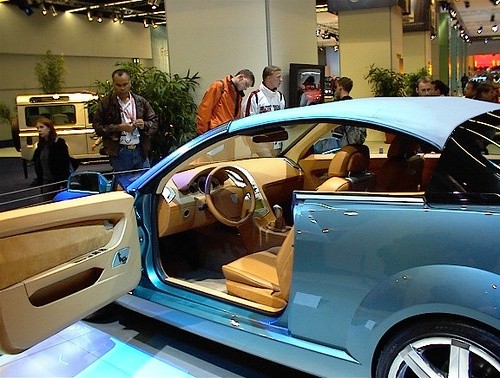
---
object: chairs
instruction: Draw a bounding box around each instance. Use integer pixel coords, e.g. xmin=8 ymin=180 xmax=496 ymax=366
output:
xmin=365 ymin=134 xmax=425 ymax=192
xmin=222 ymin=143 xmax=375 ymax=310
xmin=28 ymin=115 xmax=45 ymax=127
xmin=51 ymin=113 xmax=69 ymax=125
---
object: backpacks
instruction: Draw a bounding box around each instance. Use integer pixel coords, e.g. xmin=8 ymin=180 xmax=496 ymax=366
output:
xmin=339 ymin=125 xmax=367 ymax=147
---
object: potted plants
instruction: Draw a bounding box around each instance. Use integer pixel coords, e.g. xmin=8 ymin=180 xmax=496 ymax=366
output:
xmin=0 ymin=104 xmax=32 ymax=152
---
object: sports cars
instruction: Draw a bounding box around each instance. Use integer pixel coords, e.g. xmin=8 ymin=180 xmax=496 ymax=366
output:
xmin=0 ymin=98 xmax=500 ymax=378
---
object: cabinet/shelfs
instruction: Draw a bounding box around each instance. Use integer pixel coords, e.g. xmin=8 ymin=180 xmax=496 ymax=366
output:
xmin=289 ymin=63 xmax=325 ymax=108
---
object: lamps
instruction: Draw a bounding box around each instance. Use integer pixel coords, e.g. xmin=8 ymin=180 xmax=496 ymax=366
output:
xmin=40 ymin=3 xmax=48 ymax=16
xmin=49 ymin=5 xmax=58 ymax=18
xmin=332 ymin=43 xmax=339 ymax=52
xmin=316 ymin=23 xmax=339 ymax=42
xmin=113 ymin=13 xmax=119 ymax=22
xmin=319 ymin=46 xmax=326 ymax=51
xmin=24 ymin=5 xmax=34 ymax=16
xmin=120 ymin=14 xmax=125 ymax=24
xmin=151 ymin=19 xmax=158 ymax=29
xmin=143 ymin=18 xmax=150 ymax=28
xmin=87 ymin=10 xmax=94 ymax=22
xmin=96 ymin=16 xmax=104 ymax=24
xmin=147 ymin=0 xmax=162 ymax=10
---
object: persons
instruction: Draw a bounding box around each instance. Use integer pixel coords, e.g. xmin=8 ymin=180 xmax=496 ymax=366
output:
xmin=197 ymin=69 xmax=255 ymax=136
xmin=336 ymin=76 xmax=354 ymax=101
xmin=240 ymin=66 xmax=286 ymax=119
xmin=32 ymin=117 xmax=75 ymax=202
xmin=300 ymin=75 xmax=323 ymax=107
xmin=415 ymin=65 xmax=500 ymax=104
xmin=91 ymin=68 xmax=159 ymax=173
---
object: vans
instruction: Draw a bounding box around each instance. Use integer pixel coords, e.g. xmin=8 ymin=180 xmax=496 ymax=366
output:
xmin=17 ymin=90 xmax=174 ymax=160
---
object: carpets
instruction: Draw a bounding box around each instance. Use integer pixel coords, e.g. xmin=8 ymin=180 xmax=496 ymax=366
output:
xmin=0 ymin=157 xmax=65 ymax=213
